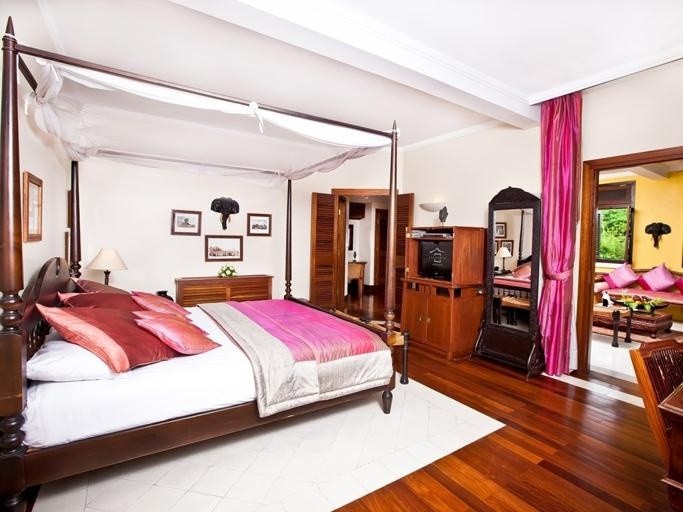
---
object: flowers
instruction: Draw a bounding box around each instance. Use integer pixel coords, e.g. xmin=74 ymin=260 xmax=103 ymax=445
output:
xmin=218 ymin=264 xmax=237 ymax=277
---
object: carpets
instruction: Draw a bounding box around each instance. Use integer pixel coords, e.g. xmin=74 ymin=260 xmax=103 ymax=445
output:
xmin=593 ymin=325 xmax=683 ymax=343
xmin=31 ymin=371 xmax=507 ymax=512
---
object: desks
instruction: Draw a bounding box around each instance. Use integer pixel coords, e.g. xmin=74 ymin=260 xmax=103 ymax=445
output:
xmin=657 ymin=381 xmax=683 ymax=512
xmin=348 ymin=262 xmax=367 ymax=305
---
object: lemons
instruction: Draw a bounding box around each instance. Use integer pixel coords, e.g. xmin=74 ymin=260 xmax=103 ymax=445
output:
xmin=628 ymin=298 xmax=634 ymax=303
xmin=635 ymin=301 xmax=642 ymax=305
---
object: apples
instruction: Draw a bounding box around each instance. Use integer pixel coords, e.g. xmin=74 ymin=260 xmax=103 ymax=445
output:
xmin=633 ymin=294 xmax=641 ymax=301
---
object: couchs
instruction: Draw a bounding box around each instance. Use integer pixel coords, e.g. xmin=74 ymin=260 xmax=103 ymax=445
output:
xmin=594 ymin=267 xmax=683 ymax=322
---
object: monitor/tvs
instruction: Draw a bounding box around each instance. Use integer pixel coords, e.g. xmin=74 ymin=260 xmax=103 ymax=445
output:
xmin=418 ymin=240 xmax=452 ymax=281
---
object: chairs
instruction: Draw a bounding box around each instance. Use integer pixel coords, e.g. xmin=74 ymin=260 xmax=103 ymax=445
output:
xmin=629 ymin=339 xmax=683 ymax=477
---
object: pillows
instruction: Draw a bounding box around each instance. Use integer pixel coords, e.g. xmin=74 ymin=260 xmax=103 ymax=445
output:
xmin=675 ymin=276 xmax=683 ymax=296
xmin=27 ymin=275 xmax=222 ymax=382
xmin=636 ymin=263 xmax=680 ymax=292
xmin=602 ymin=262 xmax=639 ymax=289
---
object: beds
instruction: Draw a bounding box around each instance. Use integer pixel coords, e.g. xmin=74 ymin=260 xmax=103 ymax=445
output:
xmin=0 ymin=17 xmax=398 ymax=512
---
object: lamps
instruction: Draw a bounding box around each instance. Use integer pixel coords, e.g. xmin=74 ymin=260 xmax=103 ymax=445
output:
xmin=495 ymin=247 xmax=512 ymax=272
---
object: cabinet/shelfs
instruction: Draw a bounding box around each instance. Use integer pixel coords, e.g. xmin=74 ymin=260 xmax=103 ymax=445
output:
xmin=400 ymin=226 xmax=487 ymax=361
xmin=175 ymin=275 xmax=273 ymax=307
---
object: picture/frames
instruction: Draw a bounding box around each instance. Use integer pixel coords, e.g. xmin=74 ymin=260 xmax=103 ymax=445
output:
xmin=23 ymin=171 xmax=43 ymax=243
xmin=495 ymin=223 xmax=513 ymax=256
xmin=349 ymin=225 xmax=353 ymax=250
xmin=171 ymin=209 xmax=202 ymax=236
xmin=205 ymin=235 xmax=243 ymax=262
xmin=248 ymin=212 xmax=271 ymax=236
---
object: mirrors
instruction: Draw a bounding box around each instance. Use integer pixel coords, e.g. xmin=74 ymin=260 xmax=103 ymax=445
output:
xmin=468 ymin=185 xmax=545 ymax=383
